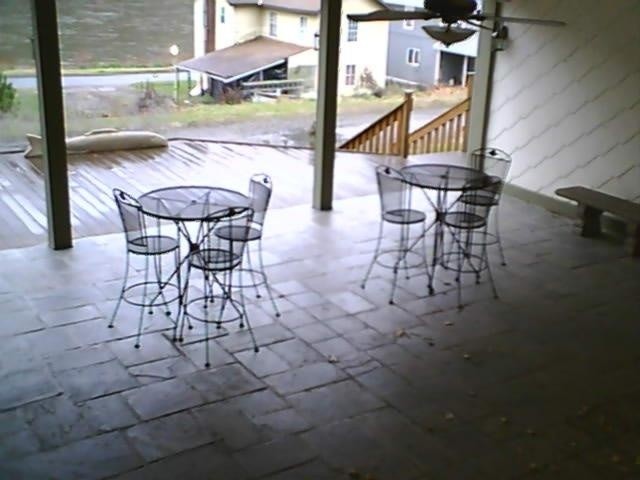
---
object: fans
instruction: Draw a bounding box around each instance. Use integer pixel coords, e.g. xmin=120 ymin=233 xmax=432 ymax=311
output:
xmin=346 ymin=0 xmax=567 ymax=34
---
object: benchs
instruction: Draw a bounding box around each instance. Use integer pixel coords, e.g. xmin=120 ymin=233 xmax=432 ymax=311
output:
xmin=554 ymin=186 xmax=640 ymax=257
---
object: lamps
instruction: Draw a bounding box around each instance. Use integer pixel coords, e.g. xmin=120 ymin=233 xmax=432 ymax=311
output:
xmin=491 ymin=26 xmax=508 ymax=52
xmin=421 ymin=16 xmax=476 ymax=48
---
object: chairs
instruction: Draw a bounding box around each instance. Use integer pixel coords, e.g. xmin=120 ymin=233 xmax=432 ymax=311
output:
xmin=360 ymin=147 xmax=512 ymax=309
xmin=108 ymin=172 xmax=281 ymax=368
